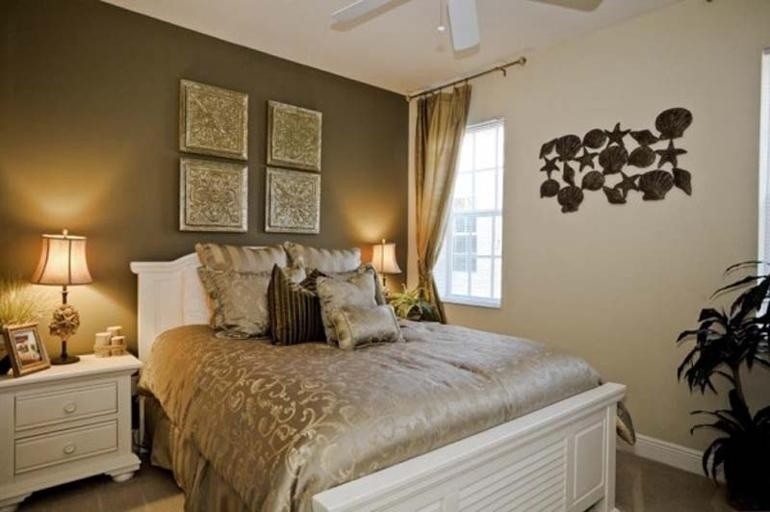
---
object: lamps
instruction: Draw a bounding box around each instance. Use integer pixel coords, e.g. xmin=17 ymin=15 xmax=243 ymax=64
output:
xmin=28 ymin=228 xmax=96 ymax=367
xmin=367 ymin=238 xmax=402 ymax=296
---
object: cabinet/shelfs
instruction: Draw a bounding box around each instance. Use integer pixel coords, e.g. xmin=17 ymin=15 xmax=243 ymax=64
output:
xmin=0 ymin=348 xmax=142 ymax=512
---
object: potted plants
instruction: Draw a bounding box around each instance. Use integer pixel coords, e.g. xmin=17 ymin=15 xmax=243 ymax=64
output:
xmin=669 ymin=259 xmax=770 ymax=507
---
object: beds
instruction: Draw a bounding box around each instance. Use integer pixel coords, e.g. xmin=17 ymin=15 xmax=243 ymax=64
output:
xmin=126 ymin=247 xmax=628 ymax=512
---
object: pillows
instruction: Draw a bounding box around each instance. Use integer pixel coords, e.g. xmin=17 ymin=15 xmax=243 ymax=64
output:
xmin=192 ymin=243 xmax=404 ymax=351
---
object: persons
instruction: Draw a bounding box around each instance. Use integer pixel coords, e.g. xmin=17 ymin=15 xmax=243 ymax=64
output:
xmin=22 ymin=344 xmax=32 ymax=360
xmin=15 ymin=343 xmax=23 ymax=360
xmin=27 ymin=344 xmax=40 ymax=360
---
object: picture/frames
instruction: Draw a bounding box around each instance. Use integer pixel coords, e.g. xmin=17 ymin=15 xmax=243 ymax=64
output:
xmin=4 ymin=323 xmax=51 ymax=377
